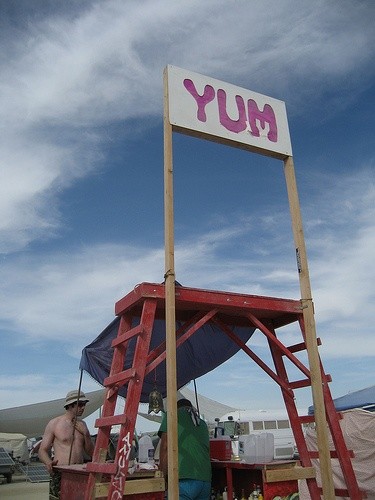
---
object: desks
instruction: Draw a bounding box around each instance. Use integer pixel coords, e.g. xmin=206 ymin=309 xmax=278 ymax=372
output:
xmin=53 ymin=464 xmax=165 ymax=500
xmin=213 ymin=460 xmax=299 ymax=500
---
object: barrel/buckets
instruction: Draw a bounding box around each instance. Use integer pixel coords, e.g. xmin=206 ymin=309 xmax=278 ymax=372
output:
xmin=238 ymin=433 xmax=274 ymax=463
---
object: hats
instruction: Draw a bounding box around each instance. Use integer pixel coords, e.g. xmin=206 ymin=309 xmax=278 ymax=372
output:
xmin=64 ymin=390 xmax=90 ymax=408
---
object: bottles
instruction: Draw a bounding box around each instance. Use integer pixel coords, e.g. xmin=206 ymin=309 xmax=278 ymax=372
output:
xmin=138 ymin=434 xmax=154 ymax=463
xmin=211 ymin=485 xmax=263 ymax=500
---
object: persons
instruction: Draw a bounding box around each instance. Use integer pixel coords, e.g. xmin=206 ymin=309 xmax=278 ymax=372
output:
xmin=128 ymin=428 xmax=139 ymax=469
xmin=157 ymin=398 xmax=213 ymax=500
xmin=37 ymin=389 xmax=93 ymax=500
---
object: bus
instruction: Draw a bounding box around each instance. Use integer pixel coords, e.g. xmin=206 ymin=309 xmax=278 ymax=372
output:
xmin=215 ymin=409 xmax=305 ymax=459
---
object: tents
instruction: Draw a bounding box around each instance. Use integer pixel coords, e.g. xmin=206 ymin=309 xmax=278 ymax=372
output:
xmin=0 ymin=281 xmax=246 ymax=440
xmin=307 ymin=385 xmax=375 ymax=416
